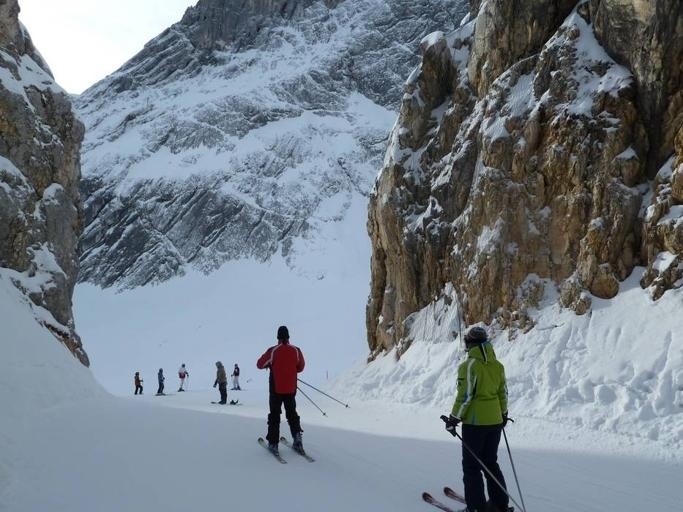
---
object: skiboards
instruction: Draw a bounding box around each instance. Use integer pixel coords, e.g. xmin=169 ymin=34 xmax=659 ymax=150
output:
xmin=151 ymin=393 xmax=176 ymax=396
xmin=227 ymin=388 xmax=246 ymax=391
xmin=258 ymin=437 xmax=315 ymax=464
xmin=211 ymin=399 xmax=238 ymax=405
xmin=422 ymin=487 xmax=514 ymax=512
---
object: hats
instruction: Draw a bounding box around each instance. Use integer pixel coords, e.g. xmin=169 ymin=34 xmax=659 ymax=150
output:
xmin=464 ymin=327 xmax=487 ymax=347
xmin=277 ymin=326 xmax=290 ymax=338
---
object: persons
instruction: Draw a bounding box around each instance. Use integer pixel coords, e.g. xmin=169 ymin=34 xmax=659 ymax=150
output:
xmin=135 ymin=372 xmax=143 ymax=395
xmin=178 ymin=363 xmax=189 ymax=391
xmin=213 ymin=362 xmax=227 ymax=404
xmin=157 ymin=368 xmax=165 ymax=394
xmin=231 ymin=364 xmax=240 ymax=390
xmin=447 ymin=328 xmax=508 ymax=511
xmin=257 ymin=326 xmax=305 ymax=452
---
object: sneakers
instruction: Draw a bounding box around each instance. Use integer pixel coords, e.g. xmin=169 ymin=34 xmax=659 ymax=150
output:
xmin=266 ymin=442 xmax=278 ymax=451
xmin=486 ymin=500 xmax=509 ymax=512
xmin=293 ymin=436 xmax=302 ymax=447
xmin=458 ymin=507 xmax=488 ymax=512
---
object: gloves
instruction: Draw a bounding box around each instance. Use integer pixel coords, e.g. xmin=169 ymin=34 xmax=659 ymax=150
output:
xmin=446 ymin=413 xmax=461 ymax=432
xmin=501 ymin=411 xmax=509 ymax=428
